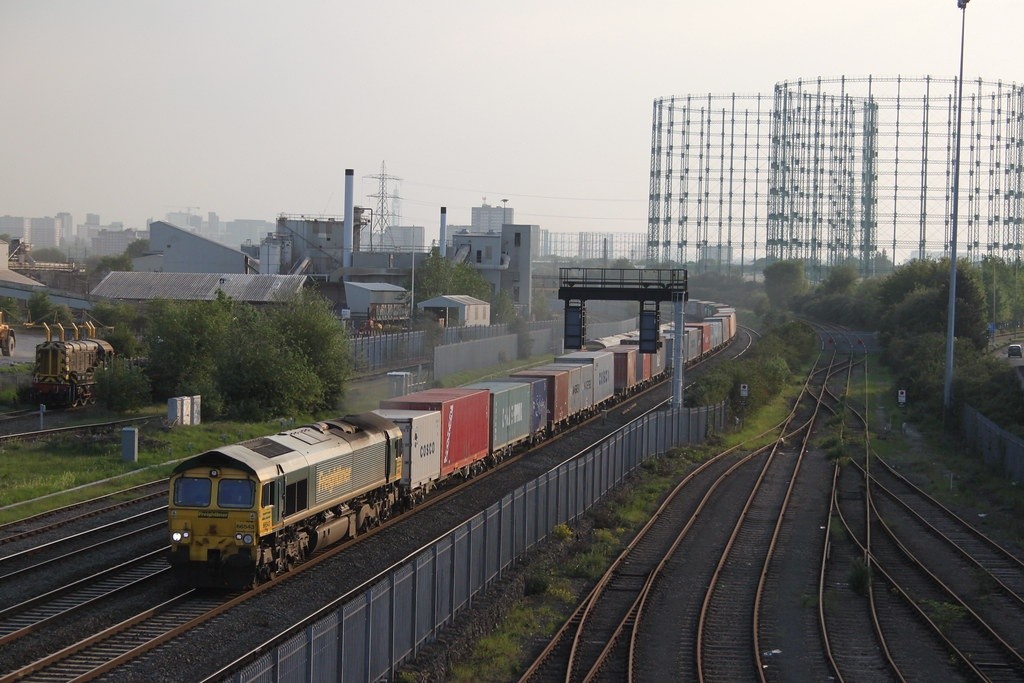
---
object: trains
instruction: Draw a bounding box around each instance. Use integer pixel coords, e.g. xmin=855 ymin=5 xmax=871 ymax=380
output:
xmin=166 ymin=297 xmax=738 ymax=595
xmin=35 ymin=337 xmax=149 ymax=408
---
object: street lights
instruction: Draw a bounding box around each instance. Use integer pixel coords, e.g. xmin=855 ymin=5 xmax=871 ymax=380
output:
xmin=500 ymin=199 xmax=508 ymax=224
xmin=945 ymin=0 xmax=968 ymax=403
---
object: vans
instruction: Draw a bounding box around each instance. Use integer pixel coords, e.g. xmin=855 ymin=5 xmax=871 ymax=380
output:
xmin=1008 ymin=344 xmax=1022 ymax=358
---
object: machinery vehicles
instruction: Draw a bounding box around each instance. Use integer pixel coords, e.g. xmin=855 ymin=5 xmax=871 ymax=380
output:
xmin=0 ymin=312 xmax=17 ymax=356
